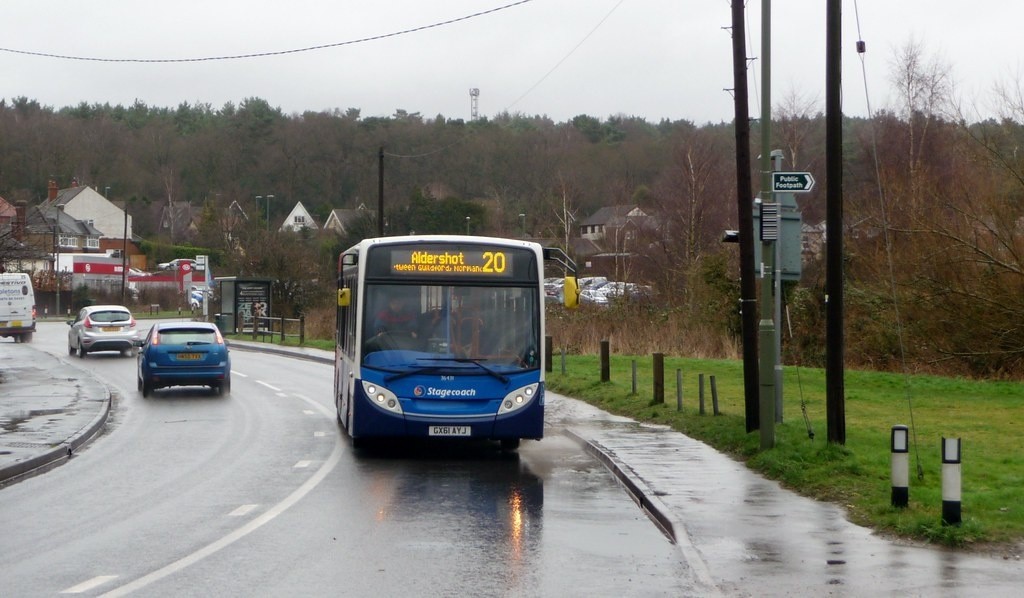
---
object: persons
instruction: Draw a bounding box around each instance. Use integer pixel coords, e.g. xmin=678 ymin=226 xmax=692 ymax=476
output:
xmin=375 ymin=294 xmax=418 ymax=339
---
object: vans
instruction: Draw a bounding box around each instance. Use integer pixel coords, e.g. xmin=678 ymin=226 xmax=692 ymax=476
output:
xmin=0 ymin=273 xmax=36 ymax=343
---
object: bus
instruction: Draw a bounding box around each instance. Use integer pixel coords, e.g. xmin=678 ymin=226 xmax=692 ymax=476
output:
xmin=334 ymin=234 xmax=580 ymax=458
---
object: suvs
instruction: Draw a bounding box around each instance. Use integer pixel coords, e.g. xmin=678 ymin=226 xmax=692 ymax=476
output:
xmin=158 ymin=258 xmax=196 ymax=272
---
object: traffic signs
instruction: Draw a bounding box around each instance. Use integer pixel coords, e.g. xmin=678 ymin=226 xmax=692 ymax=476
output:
xmin=771 ymin=170 xmax=815 ymax=193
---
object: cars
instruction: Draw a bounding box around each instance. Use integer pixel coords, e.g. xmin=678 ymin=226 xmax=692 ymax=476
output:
xmin=133 ymin=320 xmax=232 ymax=400
xmin=544 ymin=276 xmax=652 ymax=307
xmin=129 ymin=266 xmax=154 ymax=277
xmin=66 ymin=306 xmax=137 ymax=358
xmin=191 ymin=286 xmax=213 ymax=310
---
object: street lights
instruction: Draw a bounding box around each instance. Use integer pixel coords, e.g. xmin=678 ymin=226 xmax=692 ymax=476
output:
xmin=104 ymin=187 xmax=111 ymax=199
xmin=518 ymin=213 xmax=525 ymax=239
xmin=466 ymin=216 xmax=470 ymax=235
xmin=254 ymin=195 xmax=263 ymax=231
xmin=757 ymin=150 xmax=784 ymax=424
xmin=53 ymin=203 xmax=66 ymax=316
xmin=266 ymin=194 xmax=275 ymax=233
